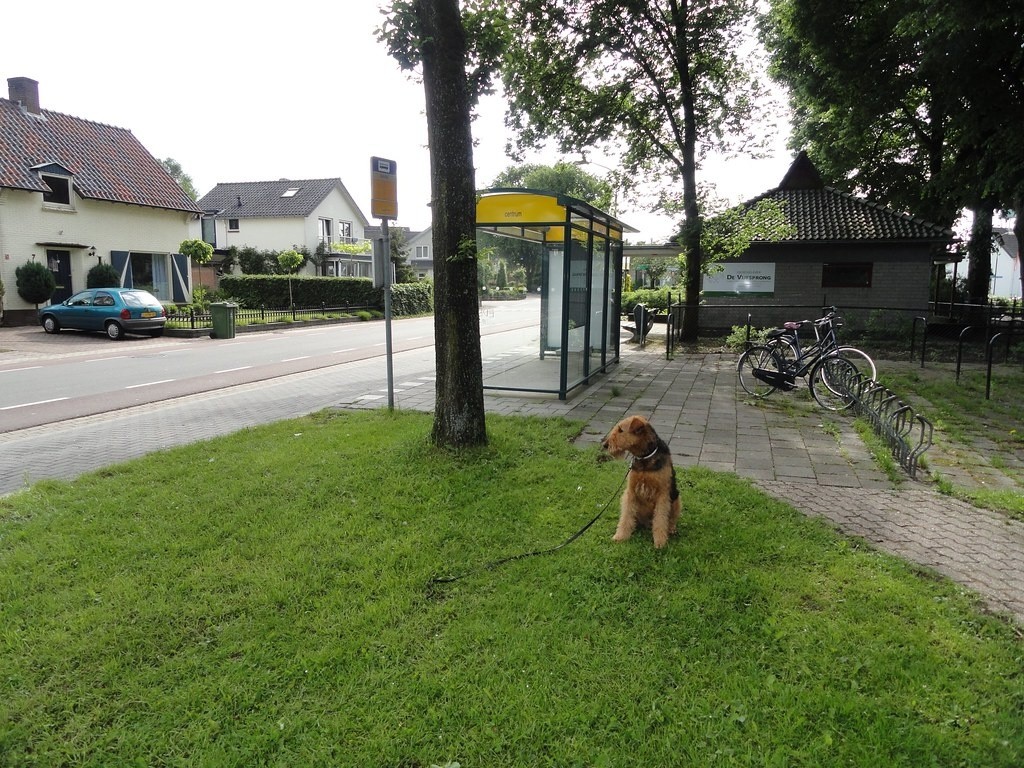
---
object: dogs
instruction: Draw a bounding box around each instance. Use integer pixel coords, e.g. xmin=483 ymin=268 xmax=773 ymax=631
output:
xmin=603 ymin=415 xmax=680 ymax=549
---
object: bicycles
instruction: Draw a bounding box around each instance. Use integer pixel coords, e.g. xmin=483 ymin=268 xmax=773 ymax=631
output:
xmin=736 ymin=306 xmax=861 ymax=411
xmin=759 ymin=317 xmax=878 ymax=398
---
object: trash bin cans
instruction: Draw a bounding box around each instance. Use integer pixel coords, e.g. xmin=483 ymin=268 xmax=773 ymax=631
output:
xmin=209 ymin=301 xmax=237 ymax=338
xmin=672 ymin=302 xmax=687 ymax=330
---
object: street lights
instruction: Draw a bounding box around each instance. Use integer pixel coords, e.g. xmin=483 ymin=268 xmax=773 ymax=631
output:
xmin=573 ymin=160 xmax=618 ymax=218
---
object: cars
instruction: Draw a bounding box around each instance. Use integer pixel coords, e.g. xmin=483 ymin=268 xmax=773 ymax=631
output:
xmin=37 ymin=287 xmax=166 ymax=340
